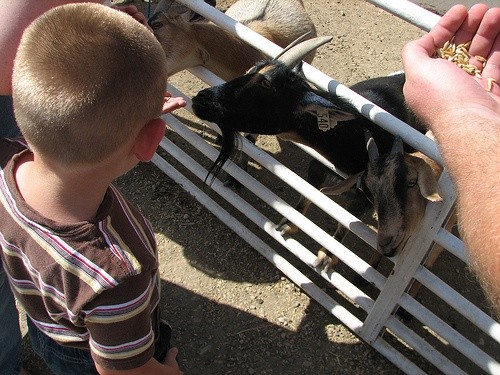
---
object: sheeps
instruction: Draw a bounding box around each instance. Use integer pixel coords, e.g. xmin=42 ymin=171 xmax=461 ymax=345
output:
xmin=190 ymin=31 xmax=432 ymax=274
xmin=318 ymin=128 xmax=458 ymax=318
xmin=146 ymin=0 xmax=317 ymax=190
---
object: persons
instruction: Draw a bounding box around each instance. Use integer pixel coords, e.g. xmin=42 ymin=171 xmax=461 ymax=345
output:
xmin=0 ymin=0 xmax=186 ymax=375
xmin=402 ymin=2 xmax=499 ymax=323
